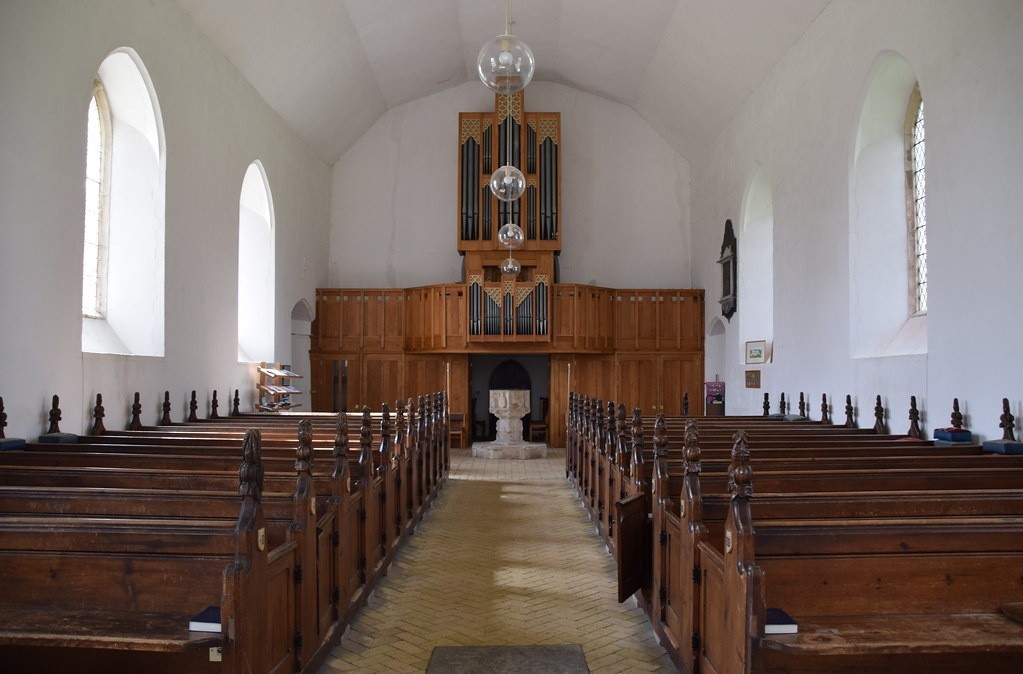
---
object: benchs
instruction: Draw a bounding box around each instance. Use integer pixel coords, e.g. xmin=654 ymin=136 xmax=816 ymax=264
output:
xmin=563 ymin=393 xmax=1023 ymax=674
xmin=0 ymin=389 xmax=450 ymax=674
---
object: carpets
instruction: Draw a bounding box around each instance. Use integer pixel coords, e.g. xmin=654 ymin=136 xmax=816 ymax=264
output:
xmin=425 ymin=644 xmax=590 ymax=674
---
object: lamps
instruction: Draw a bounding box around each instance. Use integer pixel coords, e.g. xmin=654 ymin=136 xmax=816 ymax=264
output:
xmin=476 ymin=0 xmax=535 ymax=280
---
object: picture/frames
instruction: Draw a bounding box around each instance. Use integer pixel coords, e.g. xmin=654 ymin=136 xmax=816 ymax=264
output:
xmin=746 ymin=340 xmax=765 ymax=364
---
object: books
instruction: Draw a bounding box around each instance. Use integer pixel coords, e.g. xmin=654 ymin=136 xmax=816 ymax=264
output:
xmin=764 ymin=608 xmax=798 ymax=634
xmin=711 ymin=401 xmax=722 ymax=404
xmin=189 ymin=606 xmax=221 ymax=633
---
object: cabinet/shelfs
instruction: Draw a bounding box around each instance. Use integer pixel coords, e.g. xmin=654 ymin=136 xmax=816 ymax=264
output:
xmin=255 ymin=362 xmax=304 ymax=412
